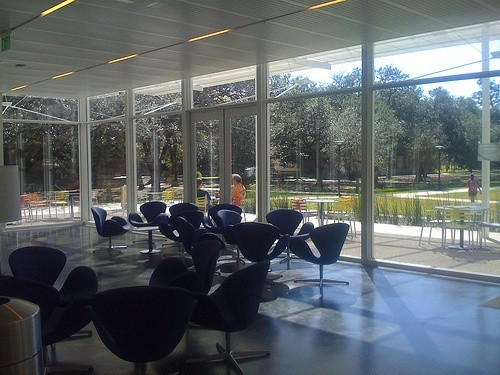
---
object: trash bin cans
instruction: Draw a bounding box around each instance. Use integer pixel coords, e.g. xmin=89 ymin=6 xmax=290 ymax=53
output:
xmin=0 ymin=296 xmax=44 ymax=375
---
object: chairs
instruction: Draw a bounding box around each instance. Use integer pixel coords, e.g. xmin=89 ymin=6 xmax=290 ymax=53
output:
xmin=0 ymin=193 xmax=357 ymax=375
xmin=415 ymin=203 xmax=500 ymax=254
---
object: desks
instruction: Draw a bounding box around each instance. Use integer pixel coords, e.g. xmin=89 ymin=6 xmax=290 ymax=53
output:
xmin=305 ymin=198 xmax=338 ymax=225
xmin=436 ymin=204 xmax=488 ymax=250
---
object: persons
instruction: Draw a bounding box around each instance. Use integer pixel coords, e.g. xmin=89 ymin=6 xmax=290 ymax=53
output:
xmin=197 ymin=178 xmax=211 ymax=211
xmin=230 ymin=174 xmax=245 ymax=206
xmin=467 ymin=174 xmax=482 ymax=202
